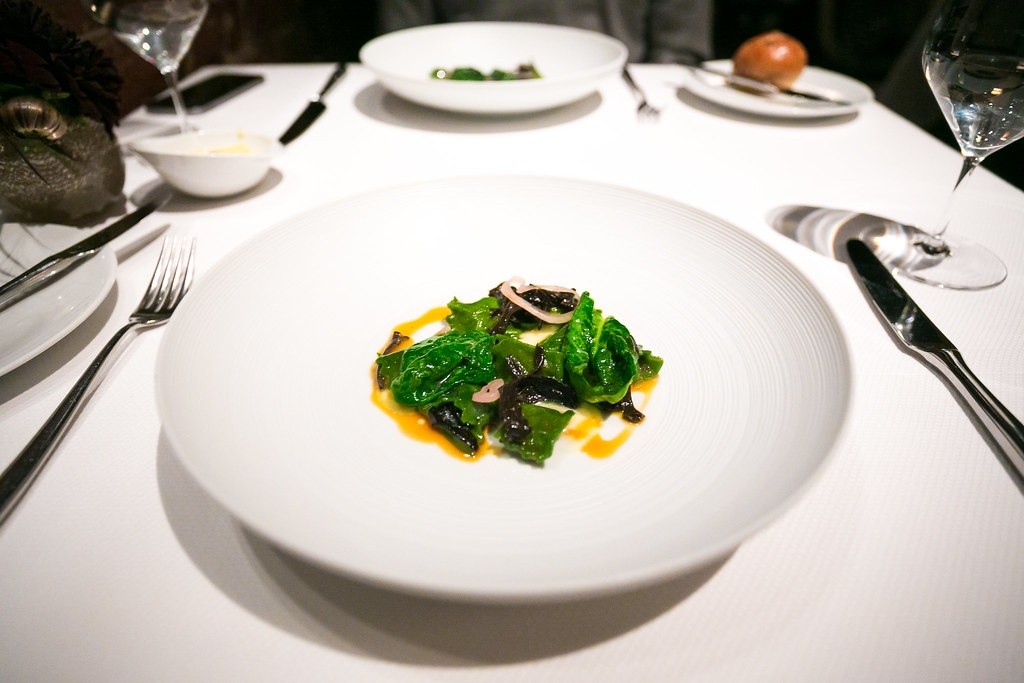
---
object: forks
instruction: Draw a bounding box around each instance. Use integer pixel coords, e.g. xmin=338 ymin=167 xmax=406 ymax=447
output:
xmin=0 ymin=233 xmax=194 ymax=528
xmin=623 ymin=69 xmax=663 ymax=115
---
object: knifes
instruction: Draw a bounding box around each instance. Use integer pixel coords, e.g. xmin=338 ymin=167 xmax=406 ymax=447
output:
xmin=689 ymin=68 xmax=849 ymax=106
xmin=280 ymin=66 xmax=347 ymax=145
xmin=0 ymin=194 xmax=172 ymax=312
xmin=846 ymin=238 xmax=1024 ymax=483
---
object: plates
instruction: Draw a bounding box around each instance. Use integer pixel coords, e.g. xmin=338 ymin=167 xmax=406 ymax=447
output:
xmin=155 ymin=173 xmax=852 ymax=608
xmin=360 ymin=21 xmax=628 ymax=114
xmin=682 ymin=58 xmax=874 ymax=117
xmin=0 ymin=223 xmax=118 ymax=377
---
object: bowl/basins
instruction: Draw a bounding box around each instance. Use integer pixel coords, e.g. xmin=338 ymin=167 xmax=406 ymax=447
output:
xmin=130 ymin=131 xmax=278 ymax=198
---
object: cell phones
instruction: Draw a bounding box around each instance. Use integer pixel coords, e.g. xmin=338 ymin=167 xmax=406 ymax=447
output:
xmin=148 ymin=74 xmax=264 ymax=114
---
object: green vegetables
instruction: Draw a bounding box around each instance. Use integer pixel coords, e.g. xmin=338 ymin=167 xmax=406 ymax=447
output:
xmin=431 ymin=62 xmax=540 ymax=81
xmin=375 ymin=290 xmax=665 ymax=467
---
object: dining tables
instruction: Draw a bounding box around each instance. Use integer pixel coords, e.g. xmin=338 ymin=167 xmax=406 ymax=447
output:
xmin=0 ymin=62 xmax=1024 ymax=683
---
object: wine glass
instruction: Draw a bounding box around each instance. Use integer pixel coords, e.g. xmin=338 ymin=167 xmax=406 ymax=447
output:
xmin=85 ymin=0 xmax=207 ymax=135
xmin=859 ymin=0 xmax=1024 ymax=290
